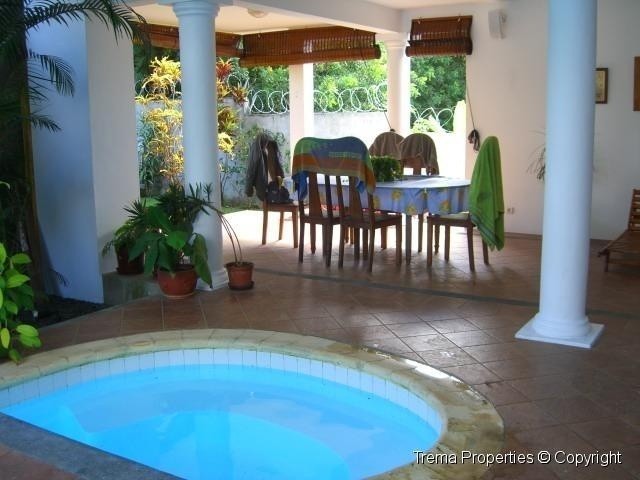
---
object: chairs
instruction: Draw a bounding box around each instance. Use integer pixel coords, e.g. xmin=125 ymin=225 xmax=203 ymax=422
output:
xmin=596 ymin=187 xmax=640 ymax=276
xmin=248 ymin=131 xmax=503 ymax=271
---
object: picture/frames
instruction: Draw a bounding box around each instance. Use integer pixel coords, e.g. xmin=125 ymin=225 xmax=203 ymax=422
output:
xmin=595 ymin=67 xmax=609 ymax=104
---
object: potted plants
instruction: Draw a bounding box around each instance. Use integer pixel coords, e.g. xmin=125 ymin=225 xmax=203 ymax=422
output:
xmin=123 ymin=181 xmax=255 ymax=290
xmin=102 ymin=219 xmax=144 ymax=275
xmin=130 ymin=207 xmax=211 ymax=299
